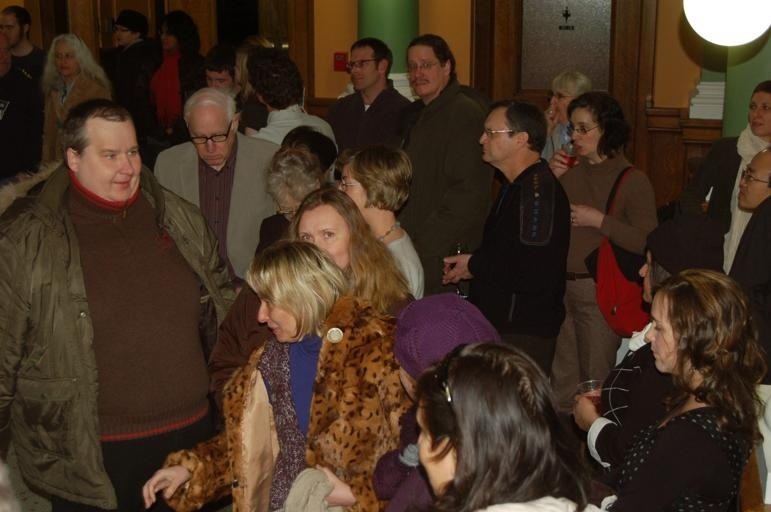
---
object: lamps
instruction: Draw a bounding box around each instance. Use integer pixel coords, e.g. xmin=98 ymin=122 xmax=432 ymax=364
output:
xmin=682 ymin=0 xmax=770 ymax=49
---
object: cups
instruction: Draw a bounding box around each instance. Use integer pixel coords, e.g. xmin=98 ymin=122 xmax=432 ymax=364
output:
xmin=577 ymin=380 xmax=606 ymax=414
xmin=562 ymin=144 xmax=579 ymax=167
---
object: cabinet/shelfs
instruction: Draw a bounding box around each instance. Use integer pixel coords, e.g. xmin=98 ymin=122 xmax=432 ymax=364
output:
xmin=677 ymin=107 xmax=722 ymax=191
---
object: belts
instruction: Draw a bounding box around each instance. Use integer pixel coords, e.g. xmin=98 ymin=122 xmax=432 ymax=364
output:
xmin=565 ymin=270 xmax=595 ymax=282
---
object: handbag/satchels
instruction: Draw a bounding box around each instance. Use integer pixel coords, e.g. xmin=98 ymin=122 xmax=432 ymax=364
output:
xmin=594 ymin=165 xmax=651 ymax=339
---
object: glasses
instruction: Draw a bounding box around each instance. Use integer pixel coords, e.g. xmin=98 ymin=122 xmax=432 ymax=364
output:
xmin=407 ymin=61 xmax=441 ymax=73
xmin=345 ymin=58 xmax=376 ymax=74
xmin=434 ymin=342 xmax=469 ymax=413
xmin=573 ymin=124 xmax=605 ymax=135
xmin=486 ymin=127 xmax=513 ymax=138
xmin=551 ymin=91 xmax=574 ymax=99
xmin=341 ymin=183 xmax=356 ymax=191
xmin=274 ymin=204 xmax=298 ymax=216
xmin=184 ymin=118 xmax=233 ymax=145
xmin=742 ymin=170 xmax=769 ymax=184
xmin=112 ymin=26 xmax=129 ymax=32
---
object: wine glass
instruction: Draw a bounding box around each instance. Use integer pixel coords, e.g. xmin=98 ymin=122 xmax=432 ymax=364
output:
xmin=448 ymin=243 xmax=468 ymax=299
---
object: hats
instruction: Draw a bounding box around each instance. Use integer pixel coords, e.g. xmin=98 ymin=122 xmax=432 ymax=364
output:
xmin=389 ymin=292 xmax=503 ymax=380
